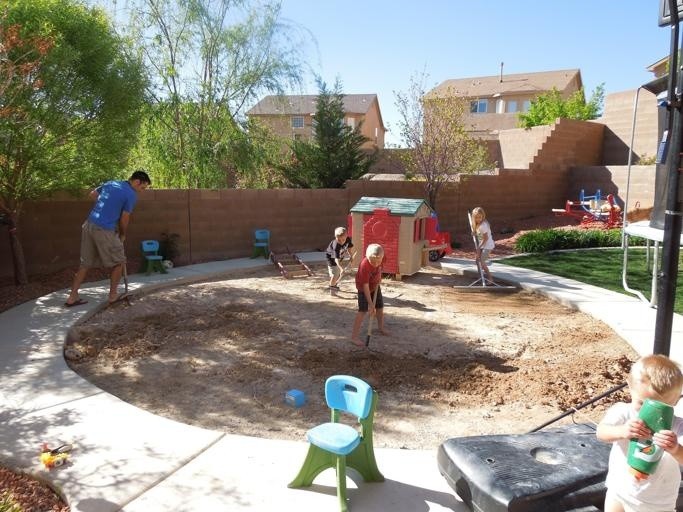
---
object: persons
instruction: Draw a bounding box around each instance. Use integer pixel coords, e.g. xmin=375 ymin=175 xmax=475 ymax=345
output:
xmin=593 ymin=353 xmax=683 ymax=512
xmin=324 ymin=226 xmax=358 ymax=298
xmin=347 ymin=242 xmax=392 ymax=347
xmin=63 ymin=170 xmax=151 ymax=307
xmin=469 ymin=205 xmax=496 ymax=287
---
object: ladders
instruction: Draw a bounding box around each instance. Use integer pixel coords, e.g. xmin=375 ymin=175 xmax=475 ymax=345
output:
xmin=270 ymin=251 xmax=316 ymax=279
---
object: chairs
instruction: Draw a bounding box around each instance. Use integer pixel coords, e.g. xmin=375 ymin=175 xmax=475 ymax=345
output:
xmin=288 ymin=374 xmax=386 ymax=512
xmin=252 ymin=229 xmax=271 ymax=259
xmin=142 ymin=240 xmax=167 ymax=274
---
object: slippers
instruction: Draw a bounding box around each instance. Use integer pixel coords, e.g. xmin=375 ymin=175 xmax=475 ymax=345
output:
xmin=64 ymin=299 xmax=88 ymax=306
xmin=109 ymin=293 xmax=133 ymax=304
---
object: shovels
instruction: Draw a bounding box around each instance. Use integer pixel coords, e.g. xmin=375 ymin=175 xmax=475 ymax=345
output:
xmin=122 ymin=243 xmax=129 ymax=306
xmin=328 ymin=251 xmax=357 ymax=290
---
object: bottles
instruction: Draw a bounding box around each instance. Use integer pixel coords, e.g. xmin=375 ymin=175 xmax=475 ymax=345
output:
xmin=626 ymin=398 xmax=673 ymax=489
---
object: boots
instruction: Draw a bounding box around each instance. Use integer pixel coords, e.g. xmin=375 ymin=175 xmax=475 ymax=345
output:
xmin=329 ymin=285 xmax=339 ymax=296
